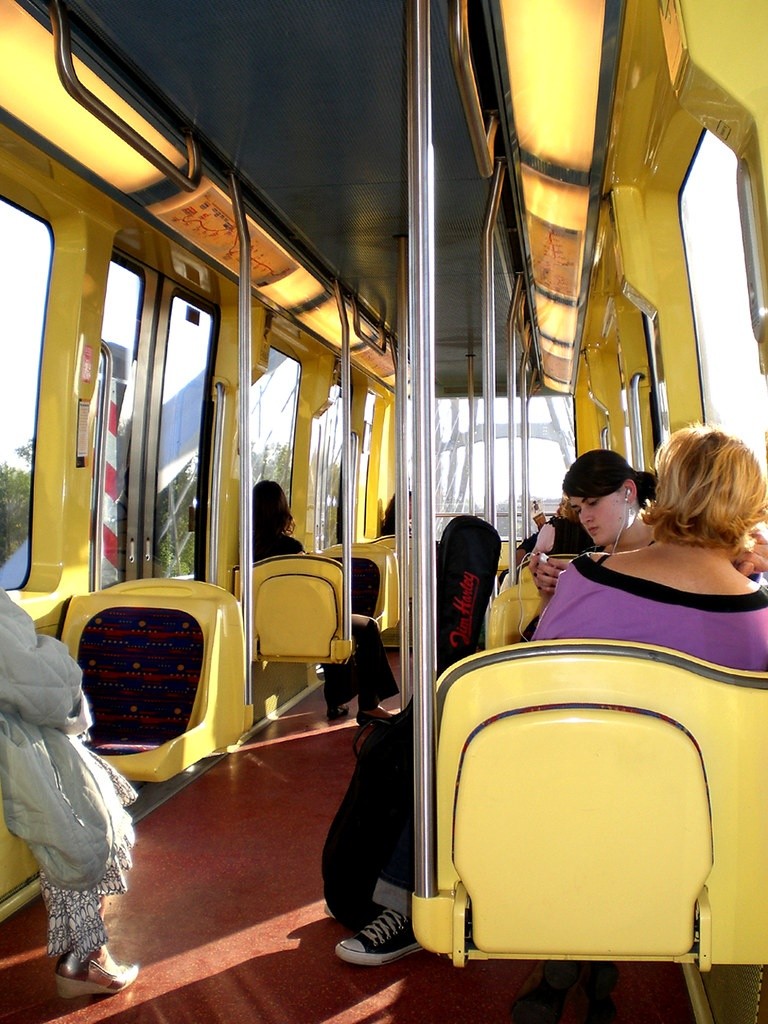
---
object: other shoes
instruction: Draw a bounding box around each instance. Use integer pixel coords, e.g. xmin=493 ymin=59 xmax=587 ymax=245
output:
xmin=327 ymin=705 xmax=350 ymax=720
xmin=356 ymin=711 xmax=399 ymax=727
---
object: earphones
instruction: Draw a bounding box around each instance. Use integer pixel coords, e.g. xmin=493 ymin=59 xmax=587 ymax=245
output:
xmin=626 ymin=489 xmax=631 ymax=501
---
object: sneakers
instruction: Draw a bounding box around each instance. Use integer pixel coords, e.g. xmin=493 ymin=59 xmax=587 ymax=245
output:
xmin=336 ymin=908 xmax=427 ymax=967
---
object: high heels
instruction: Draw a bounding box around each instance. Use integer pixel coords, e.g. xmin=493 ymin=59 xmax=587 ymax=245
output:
xmin=54 ymin=949 xmax=139 ymax=1000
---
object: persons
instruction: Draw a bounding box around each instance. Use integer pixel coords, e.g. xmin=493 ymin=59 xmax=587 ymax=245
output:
xmin=0 ymin=585 xmax=139 ymax=998
xmin=249 ymin=421 xmax=768 ymax=966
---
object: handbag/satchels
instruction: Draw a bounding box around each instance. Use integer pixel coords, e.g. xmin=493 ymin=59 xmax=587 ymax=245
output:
xmin=323 ymin=695 xmax=415 ymax=932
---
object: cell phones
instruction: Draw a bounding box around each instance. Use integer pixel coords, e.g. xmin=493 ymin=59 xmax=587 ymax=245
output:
xmin=538 ymin=553 xmax=549 ymax=564
